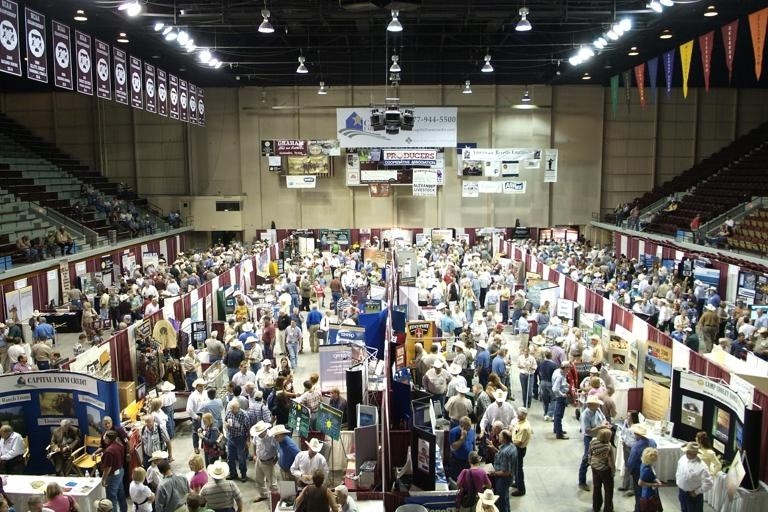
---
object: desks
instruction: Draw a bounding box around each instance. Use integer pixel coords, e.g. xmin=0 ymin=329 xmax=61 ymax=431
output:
xmin=603 ymin=367 xmax=637 ymax=419
xmin=632 ymin=417 xmax=682 ymax=483
xmin=704 ymin=462 xmax=767 ymax=510
xmin=36 ymin=310 xmax=84 ymax=334
xmin=0 ymin=471 xmax=105 ymax=512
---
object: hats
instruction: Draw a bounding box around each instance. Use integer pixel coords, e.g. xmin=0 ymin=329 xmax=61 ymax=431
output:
xmin=32 ymin=309 xmax=41 ymax=316
xmin=680 ymin=441 xmax=703 ymax=455
xmin=531 ymin=315 xmax=601 ymax=375
xmin=119 ymin=294 xmax=130 ymax=302
xmin=209 ymin=291 xmax=276 ymax=349
xmin=94 ymin=498 xmax=113 ymax=512
xmin=268 ymin=424 xmax=291 ymax=437
xmin=491 ymin=389 xmax=508 ymax=403
xmin=147 ymin=450 xmax=169 ymax=463
xmin=757 ymin=327 xmax=768 ymax=333
xmin=593 ymin=258 xmax=718 ymax=312
xmin=477 ymin=489 xmax=500 ymax=506
xmin=254 ymin=391 xmax=263 ymax=399
xmin=631 ymin=423 xmax=649 ymax=437
xmin=206 ymin=460 xmax=230 ymax=480
xmin=454 ymin=382 xmax=471 ymax=394
xmin=584 ymin=394 xmax=605 ymax=406
xmin=305 ymin=437 xmax=324 ymax=453
xmin=250 ymin=420 xmax=272 ymax=436
xmin=161 ymin=381 xmax=176 ymax=391
xmin=192 ymin=378 xmax=207 ymax=387
xmin=262 ymin=358 xmax=271 ymax=366
xmin=133 ymin=252 xmax=185 ymax=269
xmin=429 ymin=301 xmax=505 ymax=377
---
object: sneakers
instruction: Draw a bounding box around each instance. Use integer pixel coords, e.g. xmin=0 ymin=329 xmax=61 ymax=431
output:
xmin=194 ymin=447 xmax=201 ymax=454
xmin=253 ymin=494 xmax=269 ymax=503
xmin=226 ymin=473 xmax=248 ymax=482
xmin=579 ymin=481 xmax=633 ymax=496
xmin=510 ymin=483 xmax=525 ymax=498
xmin=543 ymin=412 xmax=569 ymax=440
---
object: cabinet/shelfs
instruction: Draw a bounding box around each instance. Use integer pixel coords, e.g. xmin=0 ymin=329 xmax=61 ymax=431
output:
xmin=116 ymin=379 xmax=136 ymax=408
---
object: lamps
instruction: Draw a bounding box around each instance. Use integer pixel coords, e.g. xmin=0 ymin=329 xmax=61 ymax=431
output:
xmin=318 ymin=79 xmax=328 ymax=95
xmin=385 ymin=44 xmax=402 ymax=103
xmin=569 ymin=17 xmax=632 ymax=67
xmin=582 ymin=71 xmax=592 ymax=81
xmin=152 ymin=18 xmax=222 ymax=69
xmin=705 ymin=6 xmax=718 ymax=18
xmin=117 ymin=2 xmax=141 ymax=18
xmin=258 ymin=4 xmax=275 ymax=33
xmin=259 ymin=84 xmax=270 ymax=106
xmin=512 ymin=86 xmax=539 ymax=109
xmin=481 ymin=47 xmax=494 ymax=73
xmin=647 ymin=0 xmax=696 ymax=16
xmin=297 ymin=48 xmax=309 ymax=73
xmin=74 ymin=6 xmax=87 ymax=22
xmin=628 ymin=45 xmax=638 ymax=57
xmin=117 ymin=30 xmax=128 ymax=43
xmin=660 ymin=27 xmax=673 ymax=41
xmin=463 ymin=78 xmax=473 ymax=94
xmin=516 ymin=7 xmax=532 ymax=32
xmin=387 ymin=8 xmax=403 ymax=32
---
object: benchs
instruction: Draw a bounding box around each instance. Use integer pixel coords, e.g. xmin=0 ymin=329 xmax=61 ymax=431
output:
xmin=0 ymin=110 xmax=178 ymax=271
xmin=606 ymin=114 xmax=767 ymax=261
